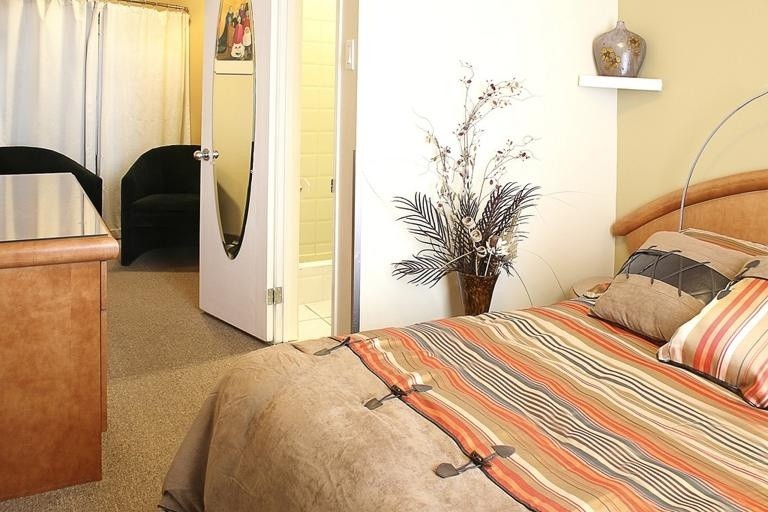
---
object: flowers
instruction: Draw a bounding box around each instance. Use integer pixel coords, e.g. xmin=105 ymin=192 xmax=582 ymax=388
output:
xmin=390 ymin=62 xmax=544 ymax=290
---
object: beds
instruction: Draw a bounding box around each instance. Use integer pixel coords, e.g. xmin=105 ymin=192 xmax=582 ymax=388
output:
xmin=157 ymin=168 xmax=767 ymax=512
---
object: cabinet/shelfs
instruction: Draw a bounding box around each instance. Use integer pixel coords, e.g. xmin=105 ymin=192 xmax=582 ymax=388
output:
xmin=0 ymin=171 xmax=120 ymax=502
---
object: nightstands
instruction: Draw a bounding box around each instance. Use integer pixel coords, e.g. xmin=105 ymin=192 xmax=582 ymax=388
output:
xmin=569 ymin=268 xmax=613 ymax=300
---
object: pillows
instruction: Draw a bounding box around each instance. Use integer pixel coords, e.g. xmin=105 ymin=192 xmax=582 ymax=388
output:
xmin=589 ymin=230 xmax=767 ymax=409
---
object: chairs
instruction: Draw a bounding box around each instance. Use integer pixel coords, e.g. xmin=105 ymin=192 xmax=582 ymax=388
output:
xmin=121 ymin=144 xmax=208 ymax=266
xmin=0 ymin=147 xmax=103 ymax=223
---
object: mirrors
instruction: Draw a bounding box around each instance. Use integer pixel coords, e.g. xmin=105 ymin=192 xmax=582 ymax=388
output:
xmin=211 ymin=0 xmax=256 ymax=260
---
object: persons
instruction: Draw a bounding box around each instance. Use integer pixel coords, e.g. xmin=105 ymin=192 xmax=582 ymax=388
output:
xmin=224 ymin=3 xmax=252 ymax=60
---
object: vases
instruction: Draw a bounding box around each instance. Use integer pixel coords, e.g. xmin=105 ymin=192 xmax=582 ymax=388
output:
xmin=455 ymin=269 xmax=502 ymax=315
xmin=593 ymin=19 xmax=648 ymax=78
